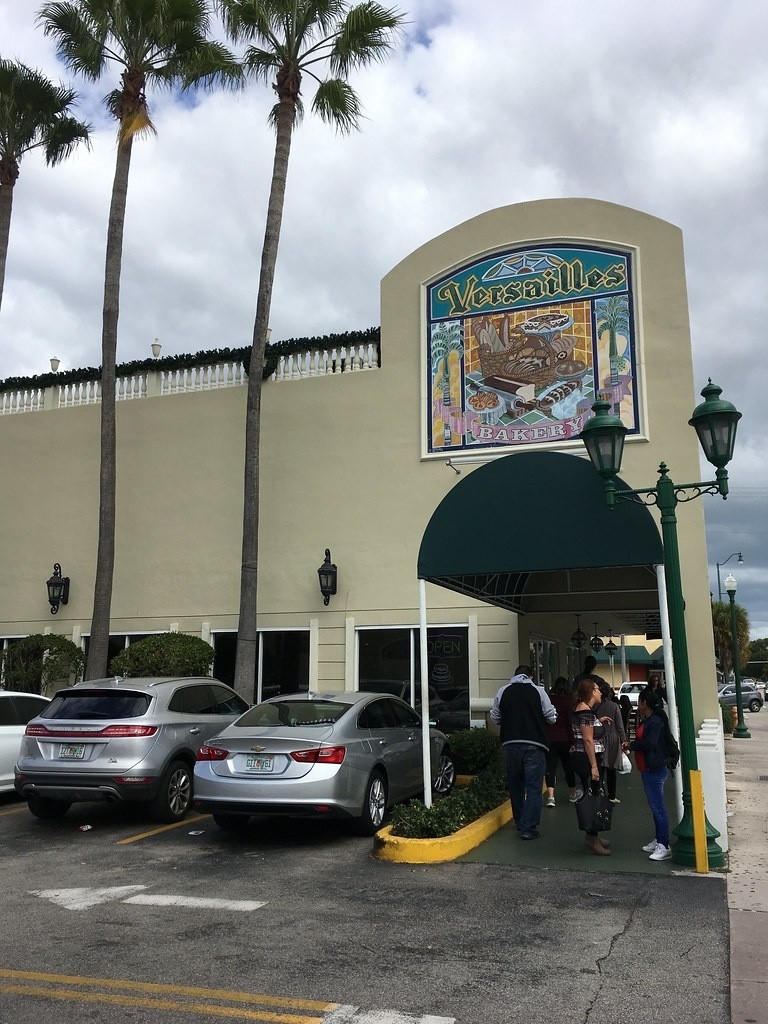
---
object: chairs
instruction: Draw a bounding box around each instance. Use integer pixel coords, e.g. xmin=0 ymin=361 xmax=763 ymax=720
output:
xmin=299 ymin=704 xmax=320 ymax=721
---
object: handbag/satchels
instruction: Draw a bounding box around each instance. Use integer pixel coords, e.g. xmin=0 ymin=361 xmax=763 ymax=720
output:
xmin=574 ymin=775 xmax=612 ymax=832
xmin=616 ymin=751 xmax=632 ymax=774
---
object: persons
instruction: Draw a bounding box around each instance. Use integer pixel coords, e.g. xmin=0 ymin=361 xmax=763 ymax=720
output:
xmin=543 ymin=655 xmax=633 ymax=855
xmin=640 ymin=674 xmax=668 ymax=708
xmin=623 ymin=690 xmax=677 ymax=860
xmin=490 ymin=664 xmax=559 ymax=839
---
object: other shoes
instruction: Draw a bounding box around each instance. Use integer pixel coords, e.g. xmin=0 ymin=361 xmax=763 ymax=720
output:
xmin=608 ymin=797 xmax=621 ymax=804
xmin=522 ymin=830 xmax=540 ymax=840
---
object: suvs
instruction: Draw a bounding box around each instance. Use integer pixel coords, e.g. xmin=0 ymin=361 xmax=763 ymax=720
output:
xmin=12 ymin=675 xmax=261 ymax=822
xmin=717 ymin=683 xmax=764 ymax=713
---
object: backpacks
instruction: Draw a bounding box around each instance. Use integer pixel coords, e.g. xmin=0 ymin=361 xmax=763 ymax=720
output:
xmin=646 ymin=714 xmax=679 ymax=775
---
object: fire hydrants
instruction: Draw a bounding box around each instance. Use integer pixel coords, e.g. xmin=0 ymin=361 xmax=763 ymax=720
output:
xmin=731 ymin=706 xmax=739 ymax=728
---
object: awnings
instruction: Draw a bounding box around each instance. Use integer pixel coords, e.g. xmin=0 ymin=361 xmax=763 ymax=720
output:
xmin=418 ymin=451 xmax=666 ymax=615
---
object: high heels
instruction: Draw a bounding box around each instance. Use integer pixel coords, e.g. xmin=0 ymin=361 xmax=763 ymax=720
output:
xmin=584 ymin=832 xmax=610 ymax=856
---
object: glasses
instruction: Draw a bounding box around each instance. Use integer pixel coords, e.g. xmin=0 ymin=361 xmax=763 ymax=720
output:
xmin=594 ymin=687 xmax=601 ymax=691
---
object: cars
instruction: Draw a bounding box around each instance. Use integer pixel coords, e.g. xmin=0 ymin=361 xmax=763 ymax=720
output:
xmin=191 ymin=692 xmax=457 ymax=836
xmin=613 ymin=680 xmax=649 ymax=718
xmin=755 ymin=681 xmax=766 ymax=689
xmin=762 ymin=682 xmax=768 ymax=701
xmin=359 ymin=678 xmax=470 ymax=730
xmin=743 ymin=679 xmax=755 ymax=687
xmin=0 ymin=689 xmax=54 ymax=797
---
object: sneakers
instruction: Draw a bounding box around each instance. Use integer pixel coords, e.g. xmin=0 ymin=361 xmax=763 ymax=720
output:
xmin=545 ymin=797 xmax=556 ymax=807
xmin=570 ymin=788 xmax=583 ymax=803
xmin=649 ymin=843 xmax=672 ymax=861
xmin=643 ymin=838 xmax=658 ymax=852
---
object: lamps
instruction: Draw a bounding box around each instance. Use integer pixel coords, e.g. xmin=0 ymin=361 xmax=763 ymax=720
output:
xmin=266 ymin=328 xmax=272 ymax=342
xmin=589 ymin=623 xmax=604 ymax=653
xmin=151 ymin=338 xmax=161 ymax=359
xmin=50 ymin=356 xmax=60 ymax=373
xmin=317 ymin=548 xmax=337 ymax=605
xmin=45 ymin=563 xmax=70 ymax=614
xmin=605 ymin=630 xmax=618 ymax=656
xmin=446 ymin=462 xmax=461 ymax=475
xmin=571 ymin=614 xmax=587 ymax=648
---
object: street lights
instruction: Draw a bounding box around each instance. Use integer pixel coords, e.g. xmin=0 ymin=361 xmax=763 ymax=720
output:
xmin=581 ymin=372 xmax=743 ymax=868
xmin=723 ymin=573 xmax=752 ymax=739
xmin=715 ymin=552 xmax=744 ymax=601
